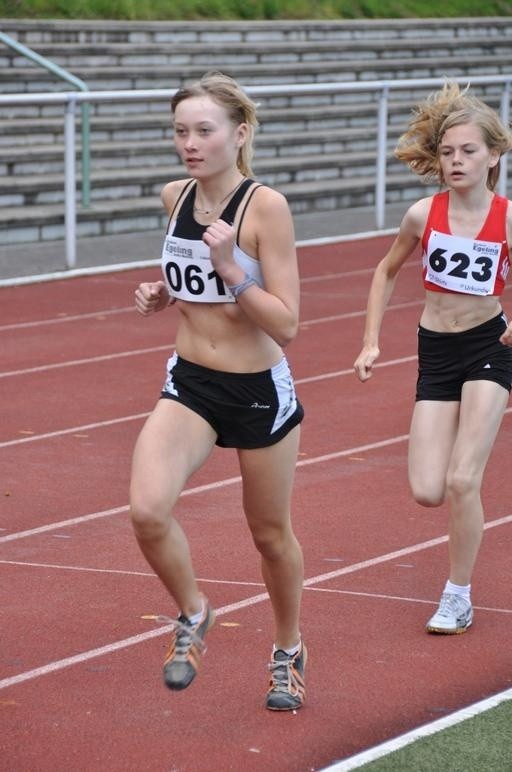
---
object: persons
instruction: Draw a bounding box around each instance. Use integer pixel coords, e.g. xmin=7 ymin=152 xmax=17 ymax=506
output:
xmin=353 ymin=72 xmax=511 ymax=636
xmin=128 ymin=70 xmax=309 ymax=711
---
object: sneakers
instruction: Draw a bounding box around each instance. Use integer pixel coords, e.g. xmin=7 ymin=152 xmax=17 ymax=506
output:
xmin=264 ymin=636 xmax=309 ymax=714
xmin=163 ymin=591 xmax=216 ymax=690
xmin=425 ymin=594 xmax=474 ymax=635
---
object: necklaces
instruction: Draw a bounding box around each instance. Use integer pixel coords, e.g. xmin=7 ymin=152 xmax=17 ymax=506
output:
xmin=195 ymin=176 xmax=247 ymax=215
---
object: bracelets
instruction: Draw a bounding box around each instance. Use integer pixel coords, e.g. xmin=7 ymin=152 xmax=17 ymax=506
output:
xmin=224 ymin=273 xmax=256 ymax=299
